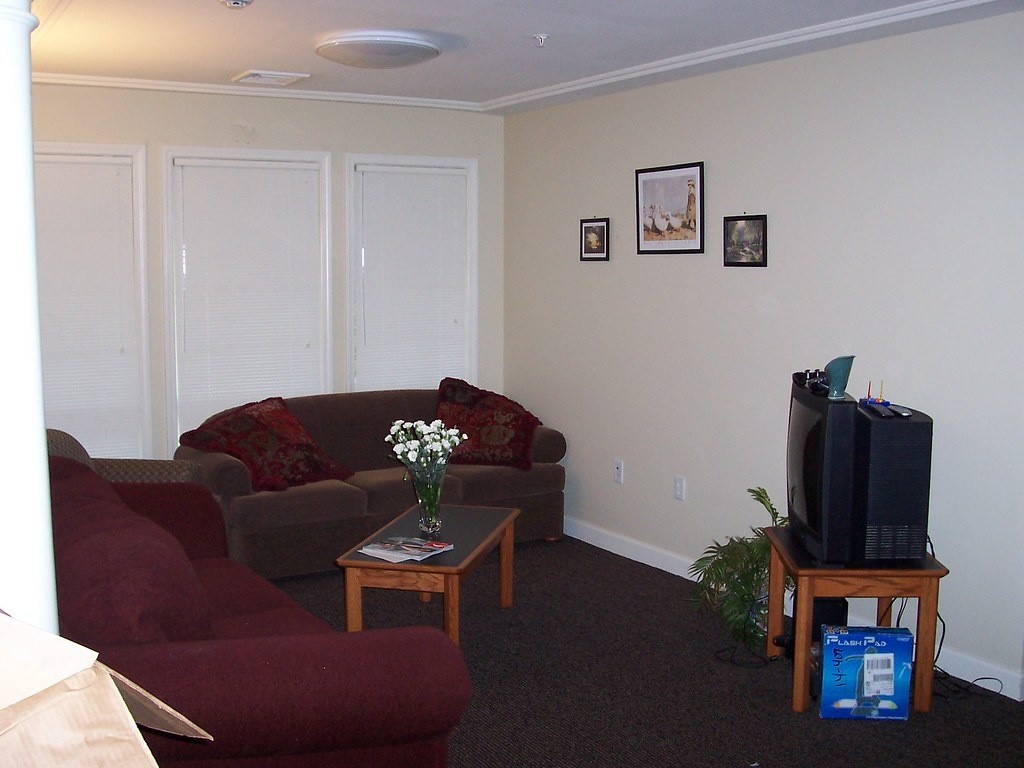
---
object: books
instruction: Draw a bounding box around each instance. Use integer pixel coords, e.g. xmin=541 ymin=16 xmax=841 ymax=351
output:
xmin=363 ymin=536 xmax=454 ymax=564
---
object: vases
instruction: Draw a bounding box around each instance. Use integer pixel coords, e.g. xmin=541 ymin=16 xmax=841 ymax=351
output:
xmin=406 ymin=467 xmax=447 ymax=533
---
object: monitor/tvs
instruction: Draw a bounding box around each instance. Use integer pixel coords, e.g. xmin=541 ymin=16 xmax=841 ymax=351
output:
xmin=786 ymin=383 xmax=934 ymax=564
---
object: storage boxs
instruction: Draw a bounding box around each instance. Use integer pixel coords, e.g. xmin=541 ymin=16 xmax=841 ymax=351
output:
xmin=817 ymin=624 xmax=914 ymax=720
xmin=0 ymin=611 xmax=215 ymax=768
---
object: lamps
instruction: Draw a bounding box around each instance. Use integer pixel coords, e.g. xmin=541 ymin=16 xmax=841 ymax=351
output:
xmin=314 ymin=31 xmax=442 ymax=69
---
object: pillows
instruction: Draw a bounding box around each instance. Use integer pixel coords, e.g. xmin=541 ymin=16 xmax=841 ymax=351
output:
xmin=435 ymin=378 xmax=543 ymax=467
xmin=180 ymin=396 xmax=355 ymax=490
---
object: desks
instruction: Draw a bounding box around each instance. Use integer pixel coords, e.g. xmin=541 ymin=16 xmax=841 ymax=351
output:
xmin=763 ymin=526 xmax=951 ymax=713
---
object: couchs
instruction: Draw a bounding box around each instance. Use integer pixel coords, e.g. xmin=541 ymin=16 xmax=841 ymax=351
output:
xmin=45 ymin=428 xmax=470 ymax=768
xmin=175 ymin=389 xmax=567 ymax=581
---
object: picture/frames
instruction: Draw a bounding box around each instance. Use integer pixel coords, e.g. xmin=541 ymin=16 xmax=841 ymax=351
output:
xmin=580 ymin=218 xmax=609 ymax=261
xmin=724 ymin=215 xmax=767 ymax=267
xmin=635 ymin=162 xmax=705 ymax=254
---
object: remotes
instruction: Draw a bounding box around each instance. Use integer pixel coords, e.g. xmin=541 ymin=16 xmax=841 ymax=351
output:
xmin=868 ymin=403 xmax=893 ymax=417
xmin=887 ymin=405 xmax=912 ymax=417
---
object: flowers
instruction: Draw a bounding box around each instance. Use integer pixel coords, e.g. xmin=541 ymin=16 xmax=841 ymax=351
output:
xmin=385 ymin=419 xmax=469 ymax=525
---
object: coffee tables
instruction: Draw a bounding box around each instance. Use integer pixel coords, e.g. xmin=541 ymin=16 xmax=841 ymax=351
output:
xmin=336 ymin=502 xmax=521 ymax=649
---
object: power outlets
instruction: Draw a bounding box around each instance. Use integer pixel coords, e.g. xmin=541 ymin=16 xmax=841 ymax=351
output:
xmin=674 ymin=476 xmax=685 ymax=500
